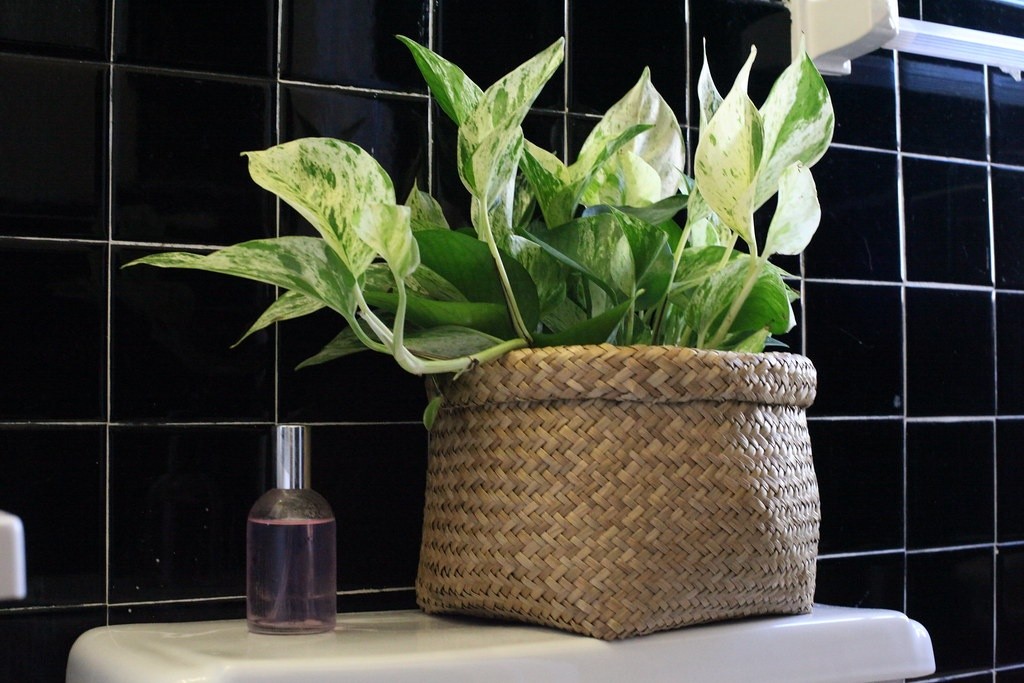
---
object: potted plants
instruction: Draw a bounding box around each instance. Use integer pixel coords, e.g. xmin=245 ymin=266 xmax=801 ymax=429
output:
xmin=119 ymin=33 xmax=823 ymax=638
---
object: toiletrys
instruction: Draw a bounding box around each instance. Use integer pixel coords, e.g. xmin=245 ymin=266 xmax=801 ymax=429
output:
xmin=243 ymin=422 xmax=342 ymax=639
